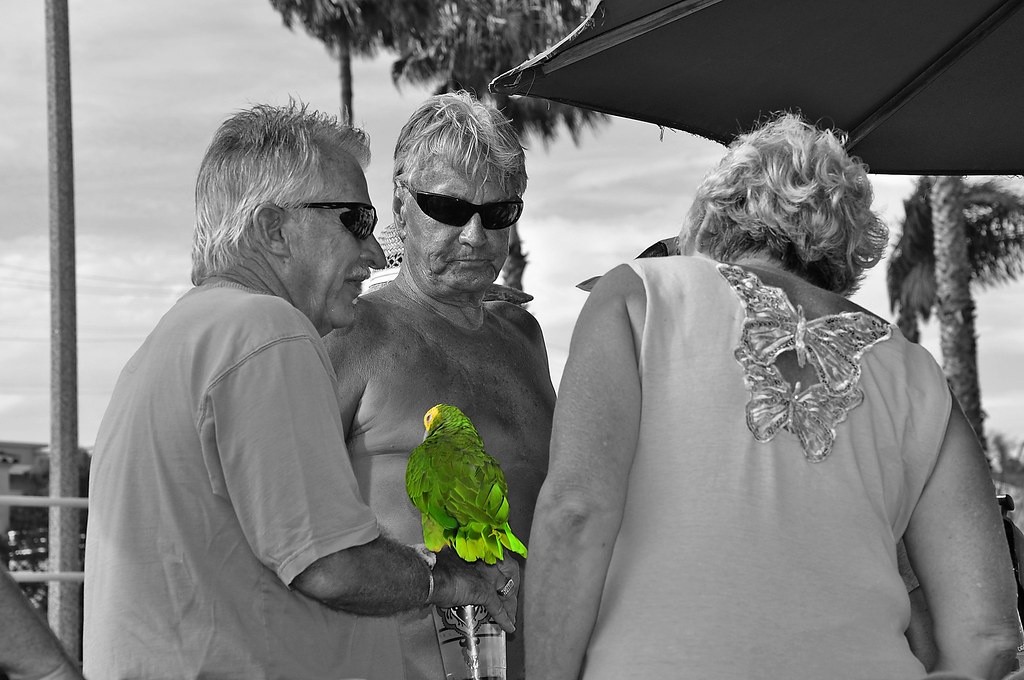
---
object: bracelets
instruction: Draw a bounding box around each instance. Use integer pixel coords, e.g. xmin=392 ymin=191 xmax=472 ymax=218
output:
xmin=425 ymin=561 xmax=434 ymax=604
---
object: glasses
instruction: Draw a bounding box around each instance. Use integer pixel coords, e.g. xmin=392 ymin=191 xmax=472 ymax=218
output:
xmin=274 ymin=201 xmax=377 ymax=241
xmin=401 ymin=179 xmax=524 ymax=230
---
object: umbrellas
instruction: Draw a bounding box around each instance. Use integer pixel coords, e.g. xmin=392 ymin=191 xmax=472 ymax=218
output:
xmin=489 ymin=0 xmax=1024 ymax=175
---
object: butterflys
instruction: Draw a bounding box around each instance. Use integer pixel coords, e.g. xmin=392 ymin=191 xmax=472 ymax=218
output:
xmin=717 ymin=262 xmax=894 ymax=464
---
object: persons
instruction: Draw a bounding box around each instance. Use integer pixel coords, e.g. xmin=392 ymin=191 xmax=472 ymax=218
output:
xmin=320 ymin=90 xmax=555 ymax=680
xmin=0 ymin=564 xmax=82 ymax=680
xmin=524 ymin=113 xmax=1021 ymax=680
xmin=84 ymin=96 xmax=519 ymax=680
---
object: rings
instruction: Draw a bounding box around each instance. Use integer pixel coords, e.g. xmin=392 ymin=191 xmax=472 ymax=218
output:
xmin=499 ymin=579 xmax=514 ymax=596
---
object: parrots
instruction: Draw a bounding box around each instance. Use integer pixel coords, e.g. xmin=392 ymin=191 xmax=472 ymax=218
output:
xmin=404 ymin=403 xmax=529 ymax=567
